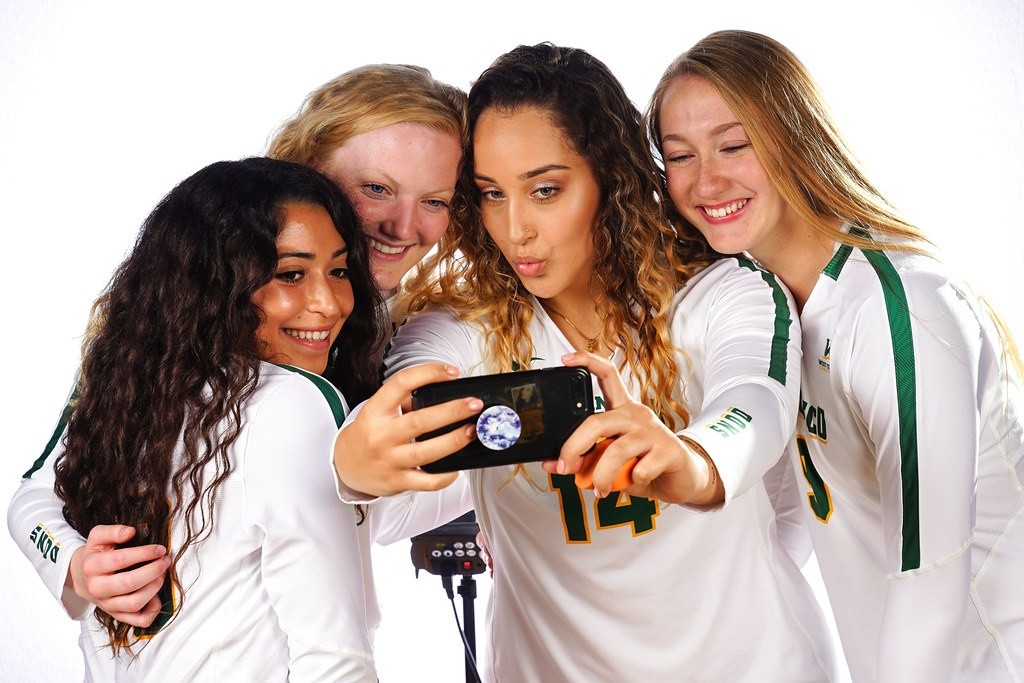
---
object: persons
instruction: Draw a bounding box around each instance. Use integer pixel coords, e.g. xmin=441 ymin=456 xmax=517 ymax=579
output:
xmin=8 ymin=61 xmax=470 ymax=627
xmin=329 ymin=40 xmax=839 ymax=683
xmin=650 ymin=30 xmax=1024 ymax=683
xmin=54 ymin=156 xmax=391 ymax=683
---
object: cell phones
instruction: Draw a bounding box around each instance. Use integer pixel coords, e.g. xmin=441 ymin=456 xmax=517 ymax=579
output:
xmin=411 ymin=366 xmax=596 ymax=474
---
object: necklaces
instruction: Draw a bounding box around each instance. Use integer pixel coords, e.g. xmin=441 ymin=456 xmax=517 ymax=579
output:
xmin=539 ymin=304 xmax=600 ymax=353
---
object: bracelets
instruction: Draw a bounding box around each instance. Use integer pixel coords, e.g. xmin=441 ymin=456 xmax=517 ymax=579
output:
xmin=677 ymin=434 xmax=717 ymax=504
